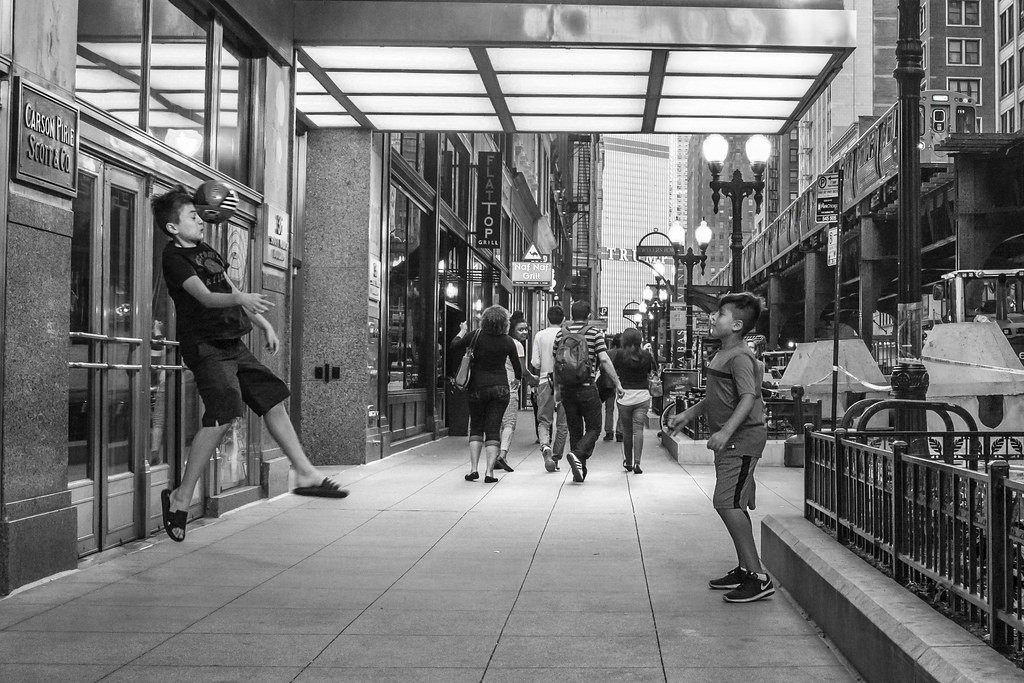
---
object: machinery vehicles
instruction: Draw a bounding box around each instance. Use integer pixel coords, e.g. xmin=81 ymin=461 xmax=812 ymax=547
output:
xmin=930 ymin=268 xmax=1024 ymax=349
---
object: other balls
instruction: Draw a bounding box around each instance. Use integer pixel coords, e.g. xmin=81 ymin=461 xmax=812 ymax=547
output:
xmin=193 ymin=180 xmax=239 ymax=225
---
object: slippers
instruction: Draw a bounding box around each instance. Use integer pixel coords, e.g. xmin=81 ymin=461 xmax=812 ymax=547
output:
xmin=292 ymin=476 xmax=351 ymax=498
xmin=161 ymin=489 xmax=188 ymax=542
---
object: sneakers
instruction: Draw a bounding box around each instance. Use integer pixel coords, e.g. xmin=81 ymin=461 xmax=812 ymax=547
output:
xmin=723 ymin=568 xmax=776 ymax=602
xmin=709 ymin=565 xmax=744 ymax=589
xmin=567 ymin=452 xmax=583 ymax=481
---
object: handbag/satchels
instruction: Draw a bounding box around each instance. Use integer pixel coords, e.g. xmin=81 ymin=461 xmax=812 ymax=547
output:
xmin=601 ymin=373 xmax=614 ymax=389
xmin=647 ymin=352 xmax=663 ymax=398
xmin=455 ymin=328 xmax=482 ymax=394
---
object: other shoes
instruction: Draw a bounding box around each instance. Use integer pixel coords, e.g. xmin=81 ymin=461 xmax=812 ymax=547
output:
xmin=623 ymin=460 xmax=632 ymax=471
xmin=494 ymin=455 xmax=514 ymax=472
xmin=549 ymin=443 xmax=551 ymax=447
xmin=540 ymin=445 xmax=555 ymax=472
xmin=465 ymin=472 xmax=479 ymax=481
xmin=552 ymin=456 xmax=560 ymax=472
xmin=536 ymin=439 xmax=540 ymax=443
xmin=616 ymin=435 xmax=623 ymax=442
xmin=603 ymin=433 xmax=613 ymax=440
xmin=485 ymin=476 xmax=498 ymax=483
xmin=634 ymin=465 xmax=642 ymax=473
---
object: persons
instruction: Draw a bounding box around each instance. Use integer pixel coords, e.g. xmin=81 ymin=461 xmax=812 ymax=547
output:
xmin=531 ymin=306 xmax=568 ymax=470
xmin=664 ymin=291 xmax=777 ymax=604
xmin=448 ymin=304 xmax=521 ymax=484
xmin=150 ymin=182 xmax=350 ymax=542
xmin=494 ymin=311 xmax=540 ymax=473
xmin=551 ymin=298 xmax=625 ymax=483
xmin=601 ymin=328 xmax=658 ymax=475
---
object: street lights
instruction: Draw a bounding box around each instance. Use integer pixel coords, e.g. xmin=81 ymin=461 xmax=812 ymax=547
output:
xmin=653 ymin=257 xmax=678 ymax=373
xmin=699 ymin=133 xmax=774 ymax=370
xmin=666 ymin=222 xmax=713 ymax=370
xmin=642 ymin=283 xmax=670 ymax=371
xmin=635 ymin=300 xmax=654 ymax=371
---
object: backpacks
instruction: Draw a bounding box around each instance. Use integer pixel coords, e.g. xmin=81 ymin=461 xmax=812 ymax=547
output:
xmin=554 ymin=325 xmax=593 ymax=388
